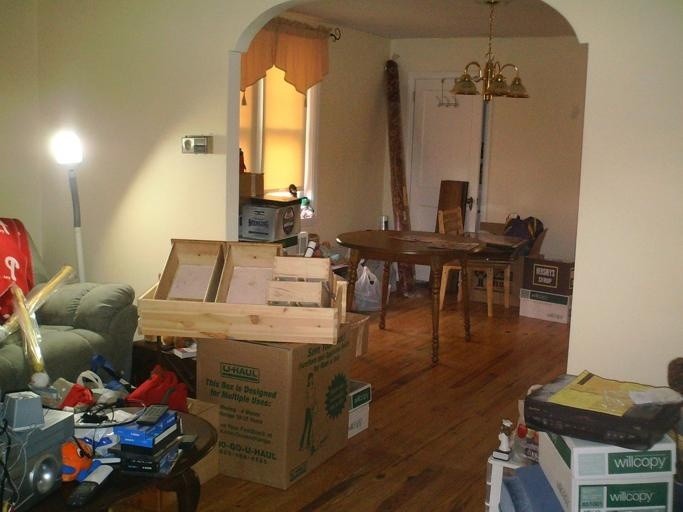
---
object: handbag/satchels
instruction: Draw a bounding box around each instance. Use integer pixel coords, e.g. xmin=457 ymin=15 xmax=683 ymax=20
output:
xmin=502 ymin=214 xmax=543 ymax=256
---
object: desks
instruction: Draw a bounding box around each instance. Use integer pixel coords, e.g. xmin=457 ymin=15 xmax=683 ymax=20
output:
xmin=335 ymin=231 xmax=486 ymax=363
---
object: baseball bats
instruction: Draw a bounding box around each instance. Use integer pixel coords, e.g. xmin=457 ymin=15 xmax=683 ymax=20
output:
xmin=5 ymin=266 xmax=73 ymax=332
xmin=13 ymin=287 xmax=45 ymax=371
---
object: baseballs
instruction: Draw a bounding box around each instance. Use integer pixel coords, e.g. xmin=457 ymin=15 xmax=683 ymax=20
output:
xmin=31 ymin=372 xmax=48 ymax=387
xmin=0 ymin=327 xmax=8 ymax=342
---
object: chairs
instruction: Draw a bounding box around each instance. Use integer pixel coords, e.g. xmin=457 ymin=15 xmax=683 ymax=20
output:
xmin=438 ymin=207 xmax=513 ymax=317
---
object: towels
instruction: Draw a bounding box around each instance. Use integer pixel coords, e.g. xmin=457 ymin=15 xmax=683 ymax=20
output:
xmin=0 ymin=218 xmax=34 ymax=320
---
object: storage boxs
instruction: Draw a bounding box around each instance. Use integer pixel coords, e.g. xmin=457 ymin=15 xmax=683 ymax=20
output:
xmin=349 ymin=380 xmax=372 ymax=440
xmin=242 ymin=203 xmax=301 ymax=248
xmin=537 ymin=432 xmax=677 ymax=479
xmin=525 ymin=259 xmax=574 ymax=295
xmin=547 ymin=479 xmax=675 ymax=512
xmin=197 ymin=312 xmax=370 ymax=490
xmin=518 ymin=288 xmax=571 ymax=324
xmin=465 ymin=222 xmax=549 ymax=307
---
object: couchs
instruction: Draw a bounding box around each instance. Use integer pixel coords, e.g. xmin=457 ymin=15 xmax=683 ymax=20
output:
xmin=0 ymin=218 xmax=138 ymax=402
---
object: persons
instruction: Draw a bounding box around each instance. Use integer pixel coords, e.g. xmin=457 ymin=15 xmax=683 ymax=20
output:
xmin=298 ymin=373 xmax=315 ymax=449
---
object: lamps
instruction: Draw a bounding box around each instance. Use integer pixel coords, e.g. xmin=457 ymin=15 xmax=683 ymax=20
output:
xmin=49 ymin=130 xmax=85 ymax=283
xmin=450 ymin=0 xmax=529 ymax=101
